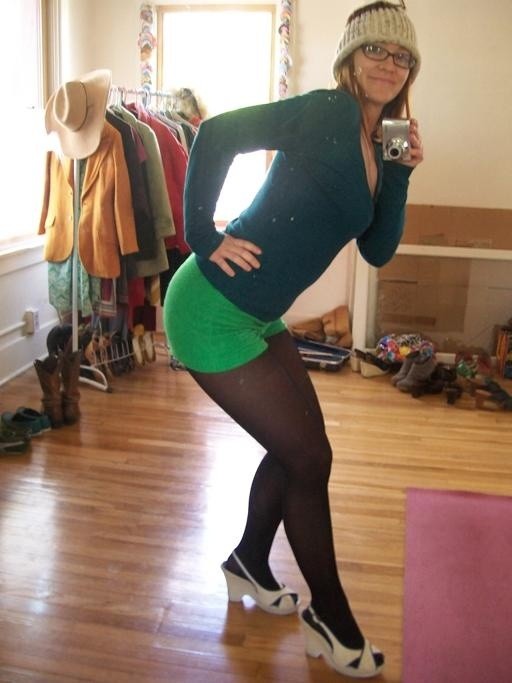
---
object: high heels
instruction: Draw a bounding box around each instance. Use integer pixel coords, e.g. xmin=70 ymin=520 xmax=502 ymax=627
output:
xmin=428 ymin=369 xmax=453 ymax=392
xmin=350 ymin=349 xmax=367 ymax=372
xmin=360 ymin=353 xmax=390 ymax=377
xmin=61 ymin=325 xmax=72 ymax=338
xmin=221 ymin=552 xmax=301 ymax=616
xmin=438 ymin=367 xmax=455 ymax=381
xmin=397 ymin=354 xmax=436 ymax=393
xmin=467 ymin=379 xmax=499 ymax=397
xmin=412 ymin=381 xmax=430 ymax=397
xmin=391 ymin=350 xmax=421 ymax=387
xmin=446 ymin=384 xmax=462 ymax=405
xmin=300 ymin=608 xmax=383 ymax=678
xmin=47 ymin=325 xmax=66 ymax=357
xmin=474 ymin=389 xmax=512 ymax=411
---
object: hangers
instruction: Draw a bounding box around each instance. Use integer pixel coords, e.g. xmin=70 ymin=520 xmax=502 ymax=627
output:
xmin=100 ymin=84 xmax=192 ymax=114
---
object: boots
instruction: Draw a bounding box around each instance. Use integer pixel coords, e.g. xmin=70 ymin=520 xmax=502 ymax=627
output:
xmin=62 ymin=348 xmax=82 ymax=425
xmin=34 ymin=356 xmax=62 ymax=428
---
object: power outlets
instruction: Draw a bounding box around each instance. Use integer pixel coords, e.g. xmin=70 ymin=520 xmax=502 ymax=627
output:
xmin=25 ymin=308 xmax=40 ymax=334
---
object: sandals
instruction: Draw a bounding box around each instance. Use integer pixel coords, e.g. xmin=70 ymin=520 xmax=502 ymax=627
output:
xmin=132 ymin=325 xmax=146 ymax=367
xmin=120 ymin=341 xmax=133 ymax=372
xmin=0 ymin=437 xmax=26 ymax=457
xmin=85 ymin=342 xmax=103 ymax=385
xmin=99 ymin=338 xmax=112 ymax=376
xmin=143 ymin=326 xmax=156 ymax=363
xmin=0 ymin=424 xmax=31 ymax=444
xmin=110 ymin=330 xmax=122 ymax=373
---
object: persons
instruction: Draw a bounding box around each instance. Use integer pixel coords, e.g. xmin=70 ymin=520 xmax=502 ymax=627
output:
xmin=162 ymin=3 xmax=425 ymax=678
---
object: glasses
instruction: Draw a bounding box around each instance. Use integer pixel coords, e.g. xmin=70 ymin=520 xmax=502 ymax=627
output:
xmin=360 ymin=44 xmax=417 ymax=69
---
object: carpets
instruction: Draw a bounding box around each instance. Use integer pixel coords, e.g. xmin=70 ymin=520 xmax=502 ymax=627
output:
xmin=399 ymin=486 xmax=512 ymax=682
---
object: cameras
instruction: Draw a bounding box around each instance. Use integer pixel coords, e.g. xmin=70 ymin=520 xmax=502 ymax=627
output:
xmin=383 ymin=119 xmax=411 ymax=161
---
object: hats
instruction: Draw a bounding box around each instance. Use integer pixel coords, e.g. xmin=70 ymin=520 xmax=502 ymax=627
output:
xmin=45 ymin=70 xmax=113 ymax=159
xmin=332 ymin=2 xmax=420 ymax=84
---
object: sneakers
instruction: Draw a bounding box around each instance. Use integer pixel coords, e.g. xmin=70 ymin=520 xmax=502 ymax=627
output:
xmin=2 ymin=412 xmax=40 ymax=437
xmin=16 ymin=406 xmax=52 ymax=433
